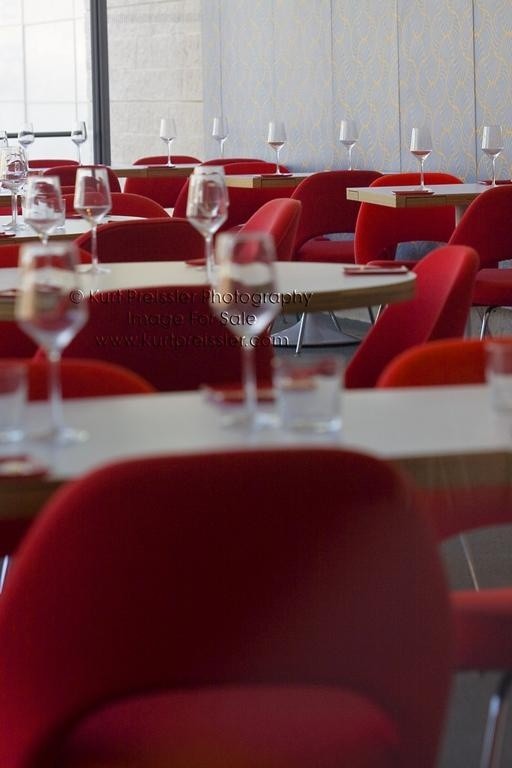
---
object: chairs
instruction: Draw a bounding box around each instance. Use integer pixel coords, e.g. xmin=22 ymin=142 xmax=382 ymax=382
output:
xmin=0 ymin=152 xmax=508 ymax=762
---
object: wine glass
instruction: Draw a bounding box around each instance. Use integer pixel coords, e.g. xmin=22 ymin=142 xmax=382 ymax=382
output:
xmin=74 ymin=168 xmax=115 ymax=273
xmin=2 ymin=120 xmax=65 ymax=243
xmin=160 ymin=117 xmax=179 ymax=166
xmin=409 ymin=126 xmax=434 ymax=190
xmin=71 ymin=120 xmax=88 ymax=165
xmin=212 ymin=115 xmax=229 ymax=164
xmin=480 ymin=124 xmax=505 ymax=189
xmin=267 ymin=120 xmax=287 ymax=175
xmin=339 ymin=117 xmax=360 ymax=170
xmin=14 ymin=241 xmax=94 ymax=445
xmin=186 ymin=163 xmax=231 ymax=262
xmin=209 ymin=229 xmax=283 ymax=430
